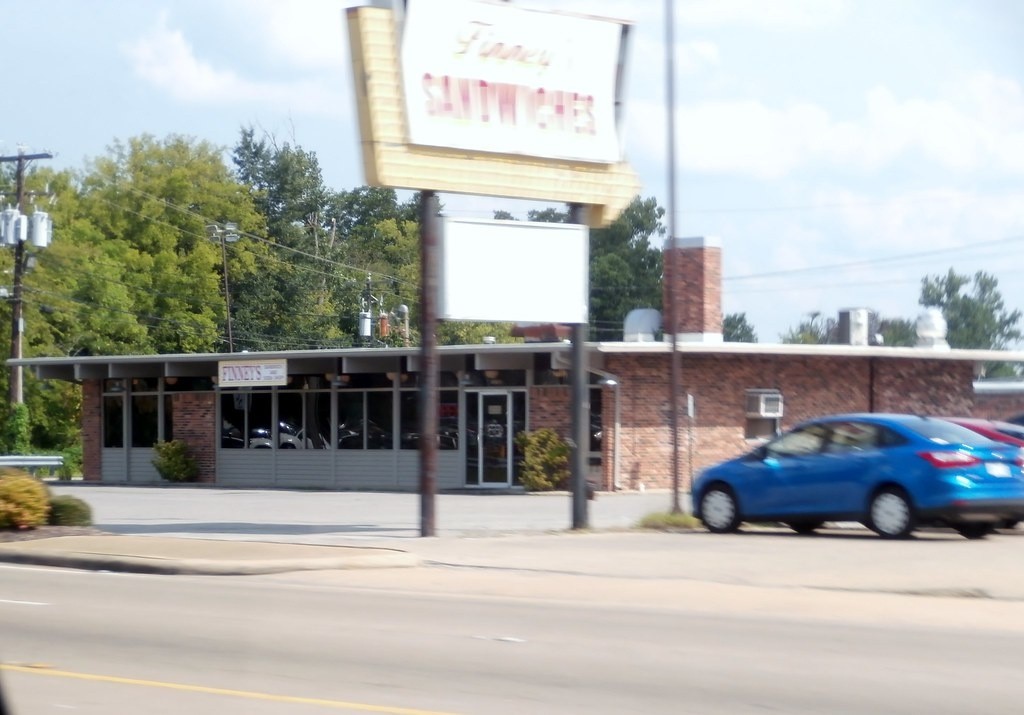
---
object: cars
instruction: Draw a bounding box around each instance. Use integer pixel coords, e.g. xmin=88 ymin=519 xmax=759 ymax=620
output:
xmin=221 ymin=415 xmax=501 ymax=450
xmin=935 ymin=415 xmax=1024 ymax=445
xmin=689 ymin=413 xmax=1024 ymax=538
